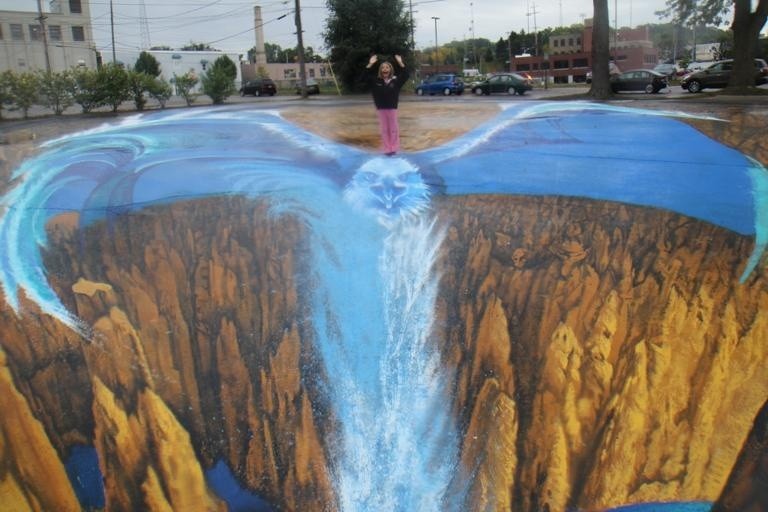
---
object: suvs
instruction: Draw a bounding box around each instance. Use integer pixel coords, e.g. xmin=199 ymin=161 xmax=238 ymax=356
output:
xmin=238 ymin=77 xmax=277 ymax=97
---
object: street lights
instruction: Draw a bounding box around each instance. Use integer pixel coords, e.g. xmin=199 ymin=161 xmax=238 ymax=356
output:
xmin=32 ymin=26 xmax=51 ymax=78
xmin=525 ymin=10 xmax=540 ymax=56
xmin=430 ymin=16 xmax=443 ymax=74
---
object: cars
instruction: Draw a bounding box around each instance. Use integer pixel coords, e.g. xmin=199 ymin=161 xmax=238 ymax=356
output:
xmin=414 ymin=67 xmax=544 ymax=98
xmin=580 ymin=57 xmax=767 ymax=96
xmin=294 ymin=77 xmax=320 ymax=94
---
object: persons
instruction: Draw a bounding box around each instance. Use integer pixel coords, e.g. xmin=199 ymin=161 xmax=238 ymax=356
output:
xmin=364 ymin=54 xmax=410 ymax=160
